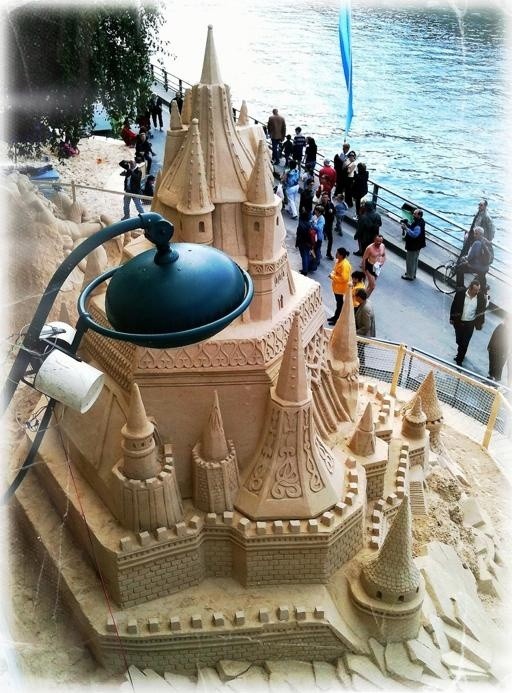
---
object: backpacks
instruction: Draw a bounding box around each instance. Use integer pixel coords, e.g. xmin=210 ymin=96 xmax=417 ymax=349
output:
xmin=309 ymin=229 xmax=318 ymax=249
xmin=479 ymin=238 xmax=494 ymax=266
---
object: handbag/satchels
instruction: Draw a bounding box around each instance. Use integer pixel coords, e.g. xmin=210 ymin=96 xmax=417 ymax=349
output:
xmin=312 ymin=192 xmax=323 ymax=204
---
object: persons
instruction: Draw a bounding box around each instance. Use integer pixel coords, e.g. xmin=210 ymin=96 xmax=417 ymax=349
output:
xmin=456 ymin=199 xmax=495 ymax=262
xmin=120 ymin=91 xmax=184 ymax=220
xmin=456 ymin=226 xmax=489 ymax=294
xmin=263 ymin=108 xmax=386 ymax=376
xmin=481 ymin=315 xmax=512 ymax=386
xmin=450 ymin=280 xmax=486 ymax=370
xmin=401 ymin=209 xmax=426 ymax=281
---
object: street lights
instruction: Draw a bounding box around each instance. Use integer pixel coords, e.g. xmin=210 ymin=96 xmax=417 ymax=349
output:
xmin=3 ymin=209 xmax=257 ymax=507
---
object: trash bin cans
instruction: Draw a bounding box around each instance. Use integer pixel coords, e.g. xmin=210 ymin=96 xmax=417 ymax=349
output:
xmin=401 ymin=203 xmax=417 ymax=241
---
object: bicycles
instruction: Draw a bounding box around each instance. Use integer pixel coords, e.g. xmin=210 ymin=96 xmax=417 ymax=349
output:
xmin=433 ymin=255 xmax=491 ymax=313
